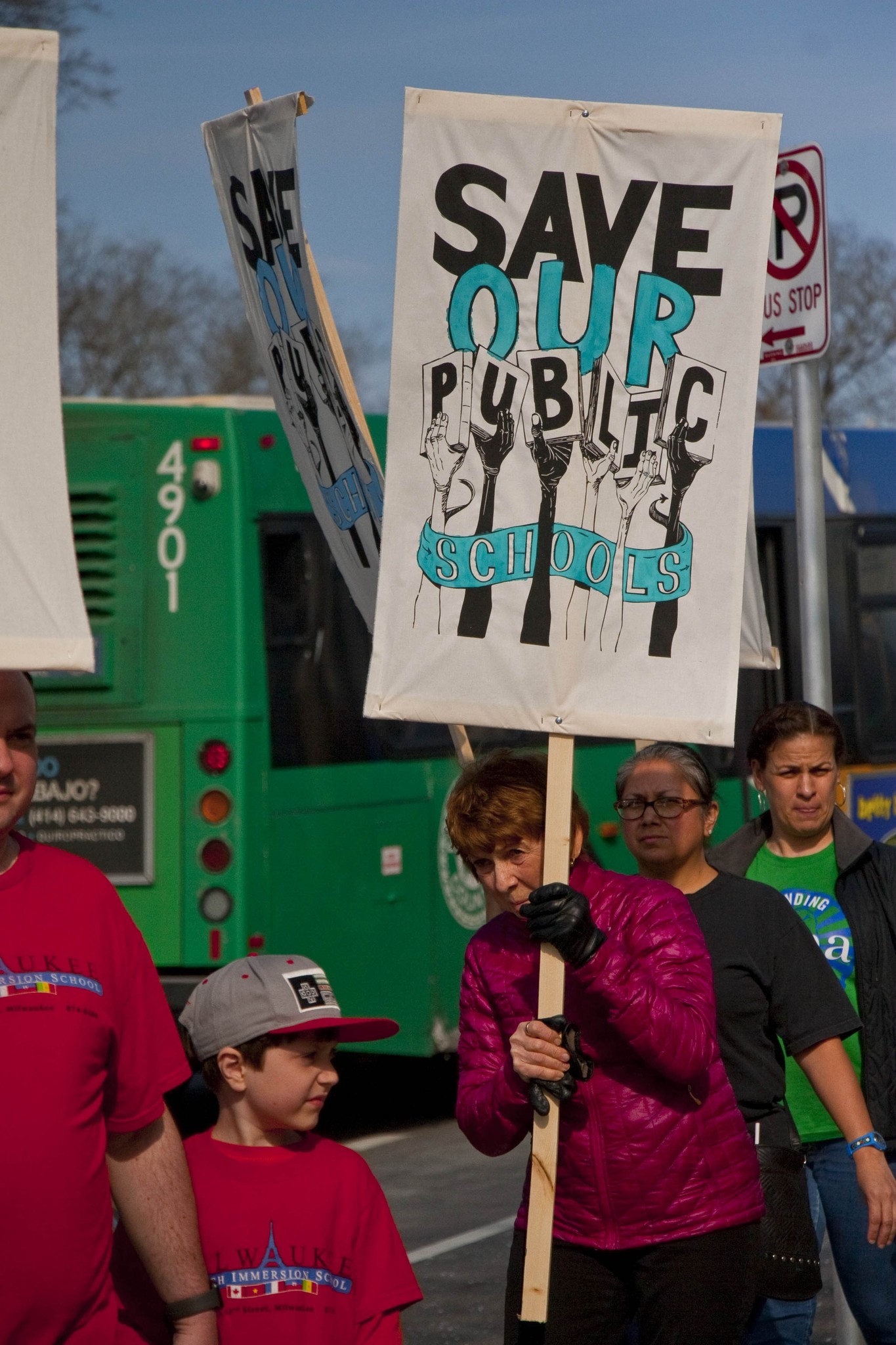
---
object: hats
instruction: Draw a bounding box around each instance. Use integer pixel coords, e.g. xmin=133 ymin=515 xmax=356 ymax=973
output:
xmin=178 ymin=952 xmax=400 ymax=1059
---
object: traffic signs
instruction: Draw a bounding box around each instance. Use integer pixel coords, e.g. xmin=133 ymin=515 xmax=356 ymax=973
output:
xmin=760 ymin=139 xmax=832 ymax=367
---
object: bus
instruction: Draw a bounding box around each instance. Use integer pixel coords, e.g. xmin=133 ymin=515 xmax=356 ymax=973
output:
xmin=12 ymin=394 xmax=895 ymax=1095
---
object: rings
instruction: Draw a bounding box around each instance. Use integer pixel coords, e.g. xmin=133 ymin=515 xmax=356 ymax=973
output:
xmin=525 ymin=1021 xmax=531 ymax=1035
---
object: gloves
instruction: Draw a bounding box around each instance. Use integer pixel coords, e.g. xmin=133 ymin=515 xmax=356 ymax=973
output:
xmin=517 ymin=882 xmax=610 ymax=968
xmin=523 ymin=1016 xmax=597 ymax=1118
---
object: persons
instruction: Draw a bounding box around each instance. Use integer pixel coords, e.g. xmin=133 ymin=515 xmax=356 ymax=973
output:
xmin=445 ymin=746 xmax=791 ymax=1345
xmin=612 ymin=742 xmax=896 ymax=1345
xmin=706 ymin=701 xmax=896 ymax=1345
xmin=111 ymin=953 xmax=425 ymax=1345
xmin=0 ymin=668 xmax=221 ymax=1344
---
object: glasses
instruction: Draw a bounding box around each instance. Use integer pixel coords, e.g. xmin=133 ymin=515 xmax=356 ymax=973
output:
xmin=613 ymin=796 xmax=709 ymax=823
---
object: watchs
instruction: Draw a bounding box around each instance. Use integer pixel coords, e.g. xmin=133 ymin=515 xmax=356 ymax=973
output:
xmin=846 ymin=1131 xmax=887 ymax=1160
xmin=165 ymin=1279 xmax=223 ymax=1320
